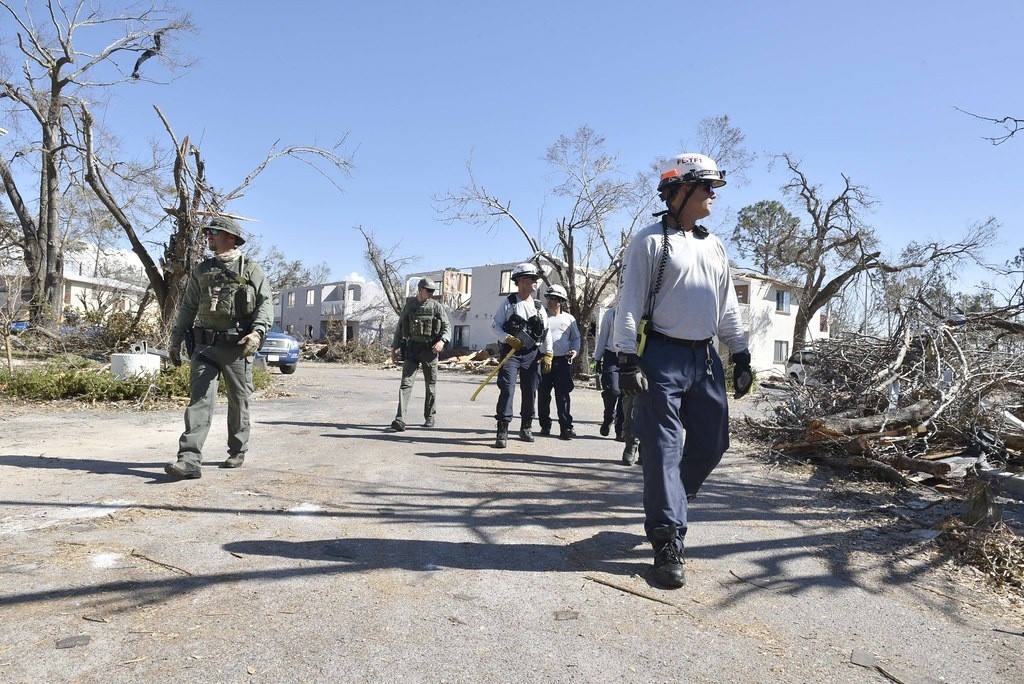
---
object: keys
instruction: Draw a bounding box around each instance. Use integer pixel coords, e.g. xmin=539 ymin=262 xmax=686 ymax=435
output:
xmin=707 ymin=365 xmax=715 ymax=381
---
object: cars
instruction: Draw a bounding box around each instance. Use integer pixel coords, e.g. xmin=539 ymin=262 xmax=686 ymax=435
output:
xmin=11 ymin=311 xmax=104 ymax=338
xmin=786 ymin=350 xmax=822 ymax=388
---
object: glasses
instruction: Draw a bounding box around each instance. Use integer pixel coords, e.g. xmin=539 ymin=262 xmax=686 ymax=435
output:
xmin=425 ymin=289 xmax=434 ymax=293
xmin=685 ymin=183 xmax=714 ymax=193
xmin=206 ymin=229 xmax=223 ymax=235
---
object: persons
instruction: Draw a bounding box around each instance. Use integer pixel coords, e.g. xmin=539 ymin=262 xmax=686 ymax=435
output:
xmin=389 ymin=277 xmax=452 ymax=432
xmin=164 ymin=216 xmax=274 ymax=477
xmin=538 ymin=284 xmax=580 ymax=438
xmin=621 ymin=396 xmax=643 ymax=464
xmin=491 ymin=262 xmax=555 ymax=446
xmin=593 ymin=306 xmax=625 ymax=442
xmin=613 ymin=153 xmax=754 ymax=585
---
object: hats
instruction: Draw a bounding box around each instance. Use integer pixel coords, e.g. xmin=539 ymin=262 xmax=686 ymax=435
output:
xmin=202 ymin=217 xmax=245 ymax=246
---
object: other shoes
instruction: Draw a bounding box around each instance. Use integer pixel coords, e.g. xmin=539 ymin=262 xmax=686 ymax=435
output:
xmin=496 ymin=431 xmax=507 ymax=448
xmin=225 ymin=452 xmax=243 ymax=468
xmin=165 ymin=461 xmax=201 ymax=478
xmin=541 ymin=427 xmax=550 ymax=434
xmin=392 ymin=420 xmax=405 ymax=431
xmin=617 ymin=432 xmax=625 ymax=441
xmin=425 ymin=417 xmax=435 ymax=427
xmin=519 ymin=428 xmax=534 ymax=442
xmin=600 ymin=419 xmax=613 ymax=435
xmin=561 ymin=427 xmax=576 ymax=439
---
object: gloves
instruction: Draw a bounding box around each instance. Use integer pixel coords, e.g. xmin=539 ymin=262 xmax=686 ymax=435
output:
xmin=236 ymin=331 xmax=260 ymax=360
xmin=169 ymin=342 xmax=181 ymax=366
xmin=506 ymin=335 xmax=522 ymax=350
xmin=618 ymin=353 xmax=649 ymax=397
xmin=536 ymin=352 xmax=553 ymax=374
xmin=732 ymin=352 xmax=753 ymax=399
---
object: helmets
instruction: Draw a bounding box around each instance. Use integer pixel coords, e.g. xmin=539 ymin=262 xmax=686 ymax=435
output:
xmin=511 ymin=263 xmax=540 ymax=281
xmin=418 ymin=279 xmax=435 ymax=289
xmin=657 ymin=153 xmax=727 ymax=192
xmin=544 ymin=284 xmax=569 ymax=302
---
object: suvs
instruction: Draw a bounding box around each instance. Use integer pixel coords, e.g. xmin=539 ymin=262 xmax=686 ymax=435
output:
xmin=255 ymin=321 xmax=300 ymax=374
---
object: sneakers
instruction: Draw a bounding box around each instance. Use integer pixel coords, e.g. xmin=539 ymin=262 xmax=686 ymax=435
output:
xmin=623 ymin=443 xmax=637 ymax=464
xmin=650 ymin=523 xmax=685 ymax=587
